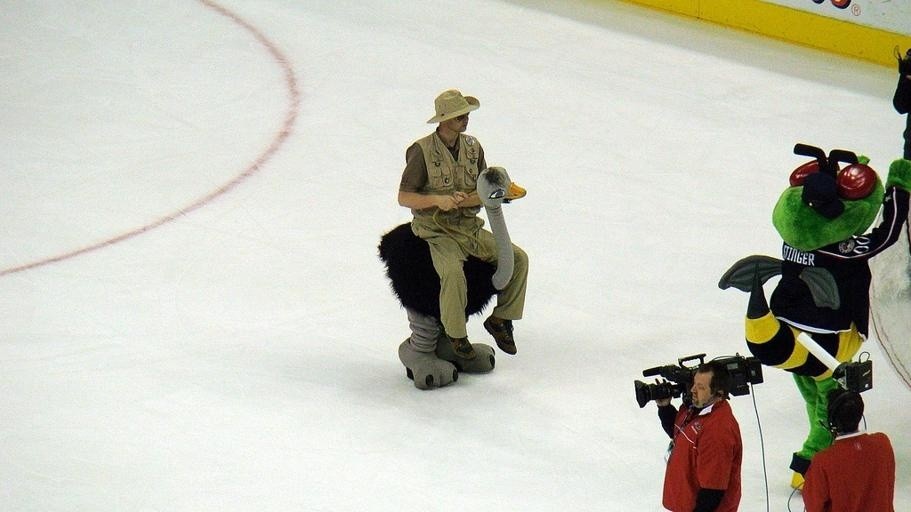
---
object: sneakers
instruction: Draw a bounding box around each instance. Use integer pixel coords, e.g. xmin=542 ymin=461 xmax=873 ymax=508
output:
xmin=446 ymin=333 xmax=477 ymax=360
xmin=483 ymin=315 xmax=517 ymax=355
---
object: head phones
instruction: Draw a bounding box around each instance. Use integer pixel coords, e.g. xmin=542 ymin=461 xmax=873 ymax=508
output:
xmin=828 ymin=391 xmax=858 ymax=433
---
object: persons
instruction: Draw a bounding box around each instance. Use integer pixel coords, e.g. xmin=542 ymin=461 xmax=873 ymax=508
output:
xmin=397 ymin=89 xmax=529 ymax=360
xmin=655 ymin=361 xmax=741 ymax=512
xmin=802 ymin=389 xmax=895 ymax=512
xmin=893 ymin=48 xmax=911 ymax=254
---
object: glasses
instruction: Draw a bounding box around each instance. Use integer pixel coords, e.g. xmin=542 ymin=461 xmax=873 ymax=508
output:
xmin=455 ymin=111 xmax=471 ymax=122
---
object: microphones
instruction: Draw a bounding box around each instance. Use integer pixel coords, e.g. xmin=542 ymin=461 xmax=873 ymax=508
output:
xmin=819 ymin=419 xmax=830 ymax=430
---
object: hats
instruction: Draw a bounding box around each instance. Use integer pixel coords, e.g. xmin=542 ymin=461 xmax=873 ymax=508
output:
xmin=426 ymin=89 xmax=480 ymax=124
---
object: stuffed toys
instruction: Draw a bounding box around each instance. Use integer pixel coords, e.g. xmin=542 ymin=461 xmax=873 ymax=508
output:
xmin=719 ymin=143 xmax=911 ymax=492
xmin=379 ymin=166 xmax=527 ymax=391
